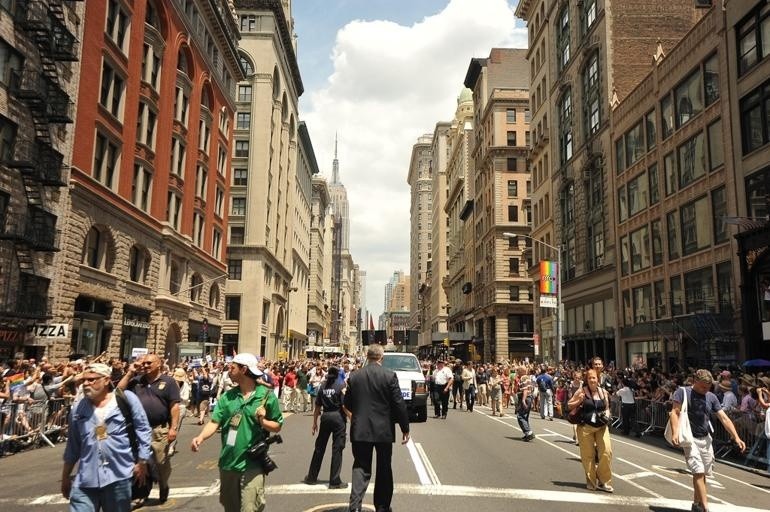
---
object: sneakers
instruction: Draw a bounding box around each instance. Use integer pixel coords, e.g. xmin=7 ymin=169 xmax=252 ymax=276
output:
xmin=541 ymin=415 xmax=554 ymax=421
xmin=159 ymin=488 xmax=169 ymax=503
xmin=622 ymin=430 xmax=641 ymax=438
xmin=48 ymin=425 xmax=60 ymax=430
xmin=691 ymin=502 xmax=703 ymax=512
xmin=596 ymin=483 xmax=613 ymax=492
xmin=720 ymin=439 xmax=732 ymax=448
xmin=199 ymin=420 xmax=204 ymax=425
xmin=521 ymin=433 xmax=535 ymax=442
xmin=283 ymin=406 xmax=308 ymax=412
xmin=493 ymin=413 xmax=503 ymax=417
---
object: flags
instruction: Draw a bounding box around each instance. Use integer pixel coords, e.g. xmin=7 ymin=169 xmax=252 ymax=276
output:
xmin=370 ymin=314 xmax=375 ymax=337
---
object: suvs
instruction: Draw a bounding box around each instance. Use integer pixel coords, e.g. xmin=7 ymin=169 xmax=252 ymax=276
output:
xmin=364 ymin=351 xmax=429 ymax=425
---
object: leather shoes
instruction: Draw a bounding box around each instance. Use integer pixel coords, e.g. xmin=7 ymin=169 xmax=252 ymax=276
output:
xmin=433 ymin=414 xmax=446 ymax=420
xmin=305 ymin=475 xmax=316 ymax=485
xmin=328 ymin=482 xmax=348 ymax=489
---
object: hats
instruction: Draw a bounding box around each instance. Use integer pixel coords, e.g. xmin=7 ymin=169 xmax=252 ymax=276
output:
xmin=758 ymin=377 xmax=770 ymax=387
xmin=740 ymin=374 xmax=752 ymax=384
xmin=231 ymin=354 xmax=262 ymax=377
xmin=720 ymin=370 xmax=731 ymax=376
xmin=193 ymin=363 xmax=202 ymax=367
xmin=80 ymin=363 xmax=112 ymax=380
xmin=719 ymin=380 xmax=732 ymax=390
xmin=173 ymin=368 xmax=186 ymax=382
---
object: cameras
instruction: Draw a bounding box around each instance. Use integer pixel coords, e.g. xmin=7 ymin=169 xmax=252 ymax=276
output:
xmin=600 ymin=411 xmax=612 ymax=425
xmin=248 ymin=443 xmax=278 ymax=475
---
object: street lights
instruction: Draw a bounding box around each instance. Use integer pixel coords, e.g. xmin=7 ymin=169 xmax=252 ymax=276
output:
xmin=502 ymin=231 xmax=562 ymax=375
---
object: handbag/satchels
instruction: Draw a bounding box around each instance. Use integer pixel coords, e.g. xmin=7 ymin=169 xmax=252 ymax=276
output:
xmin=566 ymin=404 xmax=583 ymax=424
xmin=310 ymin=387 xmax=318 ymax=397
xmin=46 ymin=399 xmax=54 ymax=416
xmin=664 ymin=411 xmax=693 ymax=447
xmin=131 ymin=464 xmax=152 ymax=499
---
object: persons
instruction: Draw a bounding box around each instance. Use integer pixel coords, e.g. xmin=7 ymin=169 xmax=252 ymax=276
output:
xmin=1 ymin=355 xmax=145 ymax=457
xmin=343 ymin=345 xmax=410 ymax=512
xmin=568 ymin=372 xmax=584 ymax=444
xmin=670 ymin=369 xmax=746 ymax=511
xmin=257 ymin=353 xmax=363 ymax=415
xmin=569 ymin=357 xmax=614 ymax=492
xmin=607 ymin=344 xmax=769 ymax=456
xmin=420 ymin=356 xmax=588 ymax=421
xmin=61 ymin=364 xmax=152 ymax=512
xmin=303 ymin=367 xmax=348 ymax=489
xmin=516 ymin=366 xmax=535 ymax=442
xmin=191 ymin=353 xmax=284 ymax=511
xmin=115 ymin=354 xmax=182 ymax=508
xmin=160 ymin=358 xmax=231 ymax=456
